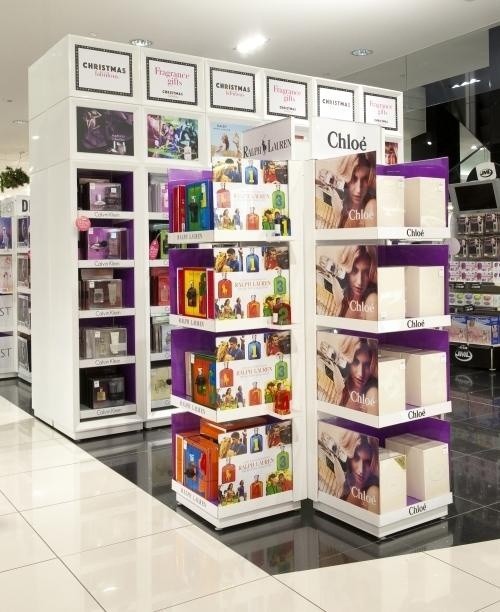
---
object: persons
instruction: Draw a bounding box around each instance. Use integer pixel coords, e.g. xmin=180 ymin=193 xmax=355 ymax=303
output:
xmin=324 ymin=244 xmax=378 ymax=322
xmin=323 ymin=150 xmax=377 ymax=228
xmin=212 ymin=133 xmax=293 ymax=507
xmin=326 ymin=430 xmax=379 ymax=514
xmin=325 ymin=335 xmax=379 ymax=416
xmin=147 ymin=117 xmax=198 ymax=160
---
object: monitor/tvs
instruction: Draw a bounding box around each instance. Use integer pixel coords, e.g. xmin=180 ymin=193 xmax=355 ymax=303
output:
xmin=448 ymin=179 xmax=500 ymax=214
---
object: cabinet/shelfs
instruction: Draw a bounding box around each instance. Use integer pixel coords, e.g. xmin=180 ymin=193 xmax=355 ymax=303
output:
xmin=165 ymin=164 xmax=294 ymax=535
xmin=313 ymin=154 xmax=455 ymax=539
xmin=1 ymin=196 xmax=31 ymax=386
xmin=28 ymin=158 xmax=191 ymax=444
xmin=448 ymin=212 xmax=500 ymax=374
